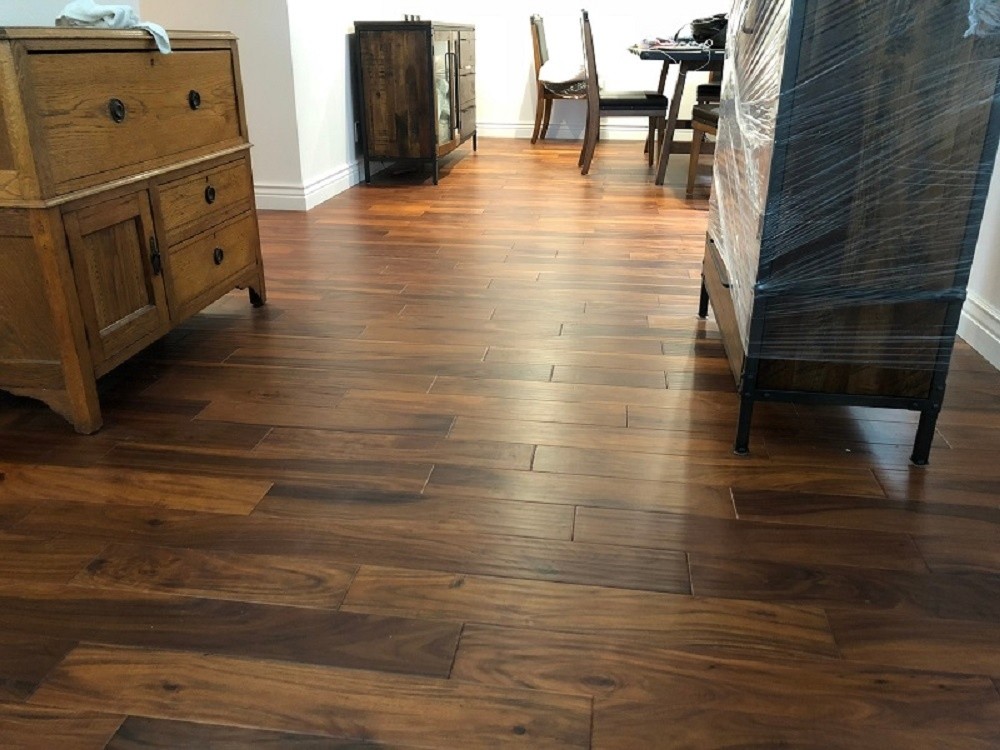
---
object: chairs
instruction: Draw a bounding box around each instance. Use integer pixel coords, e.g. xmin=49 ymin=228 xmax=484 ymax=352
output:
xmin=529 ymin=14 xmax=600 ymax=145
xmin=578 ymin=7 xmax=668 ymax=175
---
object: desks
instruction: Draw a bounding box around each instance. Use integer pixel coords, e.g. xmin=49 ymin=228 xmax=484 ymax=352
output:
xmin=628 ymin=46 xmax=725 ymax=185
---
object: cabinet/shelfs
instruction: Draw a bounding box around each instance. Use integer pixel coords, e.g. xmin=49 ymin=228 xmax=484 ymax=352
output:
xmin=0 ymin=39 xmax=267 ymax=435
xmin=697 ymin=0 xmax=1000 ymax=462
xmin=354 ymin=20 xmax=477 ymax=185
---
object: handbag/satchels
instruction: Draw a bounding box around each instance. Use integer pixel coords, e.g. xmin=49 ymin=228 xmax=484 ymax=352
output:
xmin=539 ymin=53 xmax=587 ymax=85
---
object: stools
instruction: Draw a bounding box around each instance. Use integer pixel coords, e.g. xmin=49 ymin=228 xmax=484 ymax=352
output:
xmin=687 ymin=83 xmax=722 ymax=199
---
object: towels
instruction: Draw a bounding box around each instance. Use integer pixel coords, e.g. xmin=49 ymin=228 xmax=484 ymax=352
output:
xmin=52 ymin=0 xmax=174 ymax=56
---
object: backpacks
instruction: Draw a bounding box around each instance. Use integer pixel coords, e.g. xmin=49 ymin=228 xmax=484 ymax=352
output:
xmin=674 ymin=13 xmax=724 ymax=45
